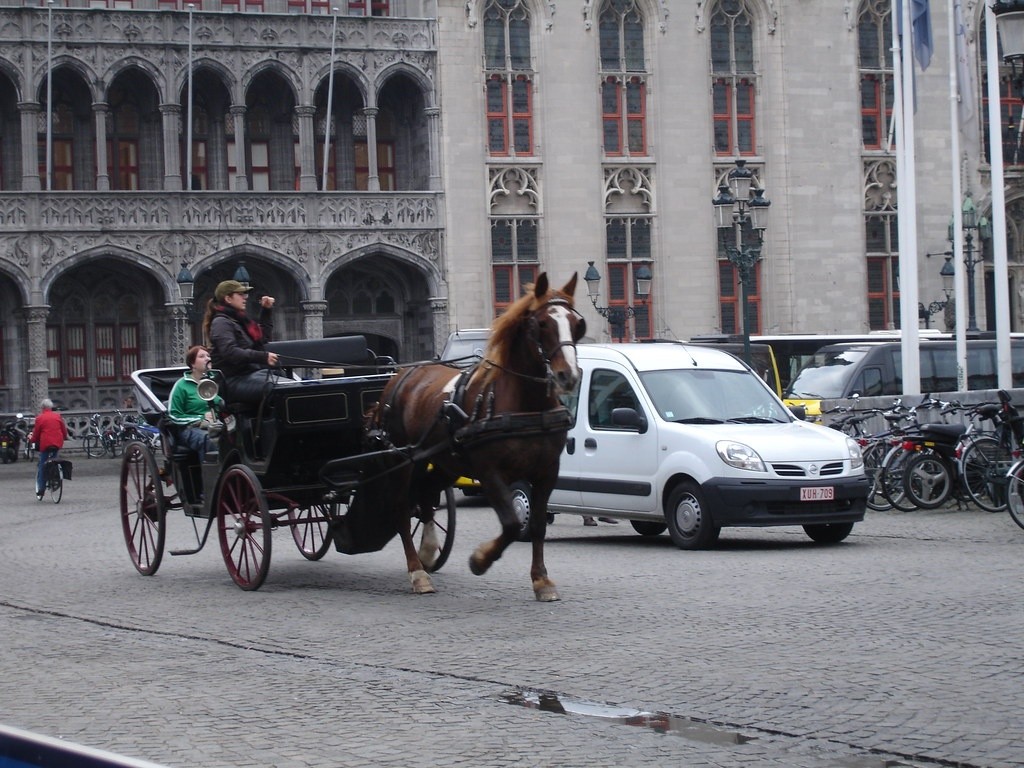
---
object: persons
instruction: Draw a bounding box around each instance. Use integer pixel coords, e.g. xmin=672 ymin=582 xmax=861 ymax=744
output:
xmin=167 ymin=345 xmax=224 ymax=502
xmin=123 ymin=397 xmax=135 ymax=409
xmin=203 ymin=279 xmax=297 ymax=400
xmin=27 ymin=399 xmax=67 ymax=495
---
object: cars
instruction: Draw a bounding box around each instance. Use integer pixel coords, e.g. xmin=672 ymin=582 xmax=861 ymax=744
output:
xmin=499 ymin=343 xmax=870 ymax=550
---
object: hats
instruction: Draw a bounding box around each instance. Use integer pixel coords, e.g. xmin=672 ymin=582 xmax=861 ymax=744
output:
xmin=215 ymin=280 xmax=254 ymax=302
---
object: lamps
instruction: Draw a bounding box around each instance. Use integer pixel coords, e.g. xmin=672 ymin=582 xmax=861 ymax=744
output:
xmin=712 ymin=156 xmax=772 ymax=285
xmin=941 ymin=192 xmax=994 ymax=302
xmin=585 ymin=259 xmax=654 ymax=339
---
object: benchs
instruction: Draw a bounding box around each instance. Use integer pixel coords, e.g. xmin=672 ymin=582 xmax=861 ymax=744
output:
xmin=161 ymin=332 xmax=367 ymax=456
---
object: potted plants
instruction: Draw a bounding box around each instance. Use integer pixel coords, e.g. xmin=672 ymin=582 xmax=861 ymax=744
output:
xmin=990 ymin=0 xmax=1024 ymax=60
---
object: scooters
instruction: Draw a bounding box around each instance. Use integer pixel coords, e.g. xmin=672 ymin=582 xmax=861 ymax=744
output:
xmin=0 ymin=413 xmax=26 ymax=464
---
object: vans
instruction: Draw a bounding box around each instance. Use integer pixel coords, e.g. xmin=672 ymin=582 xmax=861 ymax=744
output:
xmin=633 ymin=338 xmax=784 ymax=412
xmin=784 ymin=332 xmax=1023 ymax=483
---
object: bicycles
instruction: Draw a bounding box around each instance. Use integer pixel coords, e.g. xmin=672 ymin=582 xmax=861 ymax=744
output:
xmin=794 ymin=389 xmax=1023 ymax=529
xmin=28 ymin=438 xmax=65 ymax=504
xmin=22 ymin=429 xmax=36 ymax=462
xmin=85 ymin=409 xmax=163 ymax=462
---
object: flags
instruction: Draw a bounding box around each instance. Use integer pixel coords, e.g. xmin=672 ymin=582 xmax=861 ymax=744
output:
xmin=911 ymin=0 xmax=934 ymax=71
xmin=583 ymin=516 xmax=618 ymax=526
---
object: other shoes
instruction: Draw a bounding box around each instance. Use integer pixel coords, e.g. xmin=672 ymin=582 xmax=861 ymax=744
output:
xmin=199 ymin=491 xmax=204 ymax=503
xmin=36 ymin=491 xmax=44 ymax=496
xmin=599 ymin=516 xmax=618 ymax=524
xmin=583 ymin=518 xmax=597 ymax=526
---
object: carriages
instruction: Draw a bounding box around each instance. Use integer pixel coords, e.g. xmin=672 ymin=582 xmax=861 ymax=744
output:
xmin=118 ymin=268 xmax=589 ymax=607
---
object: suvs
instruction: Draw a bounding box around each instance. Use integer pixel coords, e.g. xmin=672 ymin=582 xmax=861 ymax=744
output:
xmin=424 ymin=327 xmax=492 ymax=495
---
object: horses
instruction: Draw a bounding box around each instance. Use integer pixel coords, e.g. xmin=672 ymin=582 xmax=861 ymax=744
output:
xmin=377 ymin=272 xmax=587 ymax=602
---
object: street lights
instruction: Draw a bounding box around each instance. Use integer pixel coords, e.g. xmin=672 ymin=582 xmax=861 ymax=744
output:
xmin=712 ymin=159 xmax=772 ymax=372
xmin=177 ymin=260 xmax=253 ymax=353
xmin=895 ymin=258 xmax=955 ymax=330
xmin=583 ymin=259 xmax=653 ymax=344
xmin=947 ymin=188 xmax=991 ymax=335
